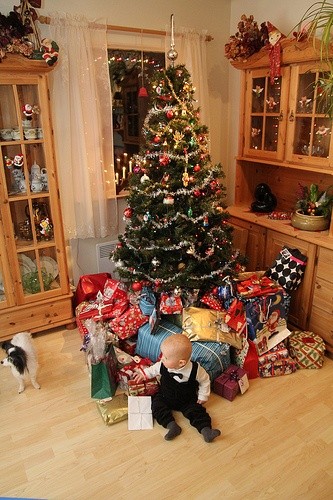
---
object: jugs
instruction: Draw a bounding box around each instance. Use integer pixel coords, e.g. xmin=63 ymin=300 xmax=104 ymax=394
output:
xmin=25 ymin=201 xmax=50 ymax=226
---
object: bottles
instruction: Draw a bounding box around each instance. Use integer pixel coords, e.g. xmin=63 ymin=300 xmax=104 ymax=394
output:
xmin=23 ymin=111 xmax=35 ymax=140
xmin=21 ymin=220 xmax=33 ymax=241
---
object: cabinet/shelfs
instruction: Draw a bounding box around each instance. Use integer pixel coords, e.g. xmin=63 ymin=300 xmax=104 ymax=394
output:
xmin=0 ymin=52 xmax=75 ymax=343
xmin=223 ymin=37 xmax=333 ymax=355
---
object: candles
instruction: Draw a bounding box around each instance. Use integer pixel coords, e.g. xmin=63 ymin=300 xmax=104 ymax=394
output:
xmin=123 ymin=169 xmax=125 ymax=178
xmin=117 ymin=161 xmax=120 ymax=169
xmin=116 ymin=173 xmax=118 ymax=184
xmin=129 ymin=163 xmax=132 ymax=172
xmin=124 ymin=156 xmax=127 ymax=163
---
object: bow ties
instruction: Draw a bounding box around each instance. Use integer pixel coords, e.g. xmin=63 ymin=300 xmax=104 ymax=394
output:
xmin=169 ymin=372 xmax=183 ymax=379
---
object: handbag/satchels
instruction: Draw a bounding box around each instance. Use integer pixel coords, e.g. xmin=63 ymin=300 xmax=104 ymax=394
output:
xmin=90 ymin=342 xmax=119 ymax=400
xmin=107 ymin=306 xmax=149 ymax=340
xmin=263 ymin=246 xmax=308 ymax=291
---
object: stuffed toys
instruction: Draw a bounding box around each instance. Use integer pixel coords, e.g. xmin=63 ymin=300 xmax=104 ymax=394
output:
xmin=12 ymin=153 xmax=23 ymax=169
xmin=22 ymin=103 xmax=34 ymax=121
xmin=41 ymin=37 xmax=59 ymax=66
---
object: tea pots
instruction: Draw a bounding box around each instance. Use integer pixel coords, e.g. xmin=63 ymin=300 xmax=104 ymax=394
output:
xmin=16 ymin=160 xmax=49 ymax=192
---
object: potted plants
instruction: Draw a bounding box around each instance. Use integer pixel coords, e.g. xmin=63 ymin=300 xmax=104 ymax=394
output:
xmin=291 ymin=184 xmax=333 ymax=231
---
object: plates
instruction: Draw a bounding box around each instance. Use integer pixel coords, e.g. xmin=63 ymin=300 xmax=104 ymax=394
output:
xmin=32 ymin=256 xmax=59 ymax=281
xmin=17 ymin=253 xmax=36 ymax=274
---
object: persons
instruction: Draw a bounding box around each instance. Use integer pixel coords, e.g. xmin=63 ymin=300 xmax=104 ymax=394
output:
xmin=132 ymin=334 xmax=221 ymax=442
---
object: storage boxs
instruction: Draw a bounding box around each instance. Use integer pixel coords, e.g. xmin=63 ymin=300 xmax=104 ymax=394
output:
xmin=75 ymin=246 xmax=326 ymax=430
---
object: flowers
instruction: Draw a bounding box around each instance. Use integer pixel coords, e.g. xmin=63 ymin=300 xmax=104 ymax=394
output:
xmin=223 ymin=15 xmax=263 ymax=61
xmin=0 ymin=11 xmax=33 ymax=62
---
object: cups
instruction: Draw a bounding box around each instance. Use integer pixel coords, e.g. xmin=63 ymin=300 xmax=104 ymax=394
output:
xmin=0 ymin=127 xmax=42 ymax=141
xmin=299 ymin=136 xmax=325 ymax=157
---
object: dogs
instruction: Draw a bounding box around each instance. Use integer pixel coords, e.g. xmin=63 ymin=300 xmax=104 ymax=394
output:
xmin=0 ymin=332 xmax=41 ymax=394
